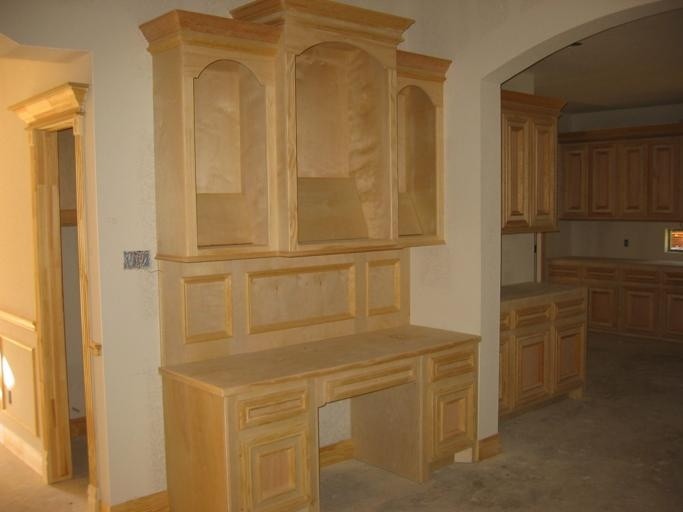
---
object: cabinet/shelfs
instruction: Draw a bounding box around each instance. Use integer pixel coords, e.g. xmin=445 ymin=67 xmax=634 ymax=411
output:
xmin=555 ymin=122 xmax=682 ymax=219
xmin=138 ymin=0 xmax=459 ymax=263
xmin=159 ymin=326 xmax=482 ymax=512
xmin=545 ymin=264 xmax=683 ymax=342
xmin=501 ymin=90 xmax=565 ymax=233
xmin=499 ymin=287 xmax=591 ymax=417
